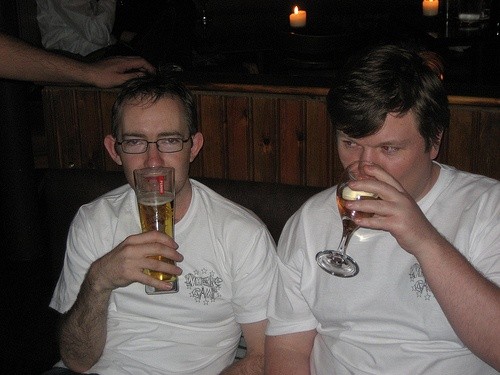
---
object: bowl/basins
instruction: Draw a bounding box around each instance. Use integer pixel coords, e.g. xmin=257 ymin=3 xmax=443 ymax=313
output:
xmin=278 ymin=22 xmax=371 ymax=55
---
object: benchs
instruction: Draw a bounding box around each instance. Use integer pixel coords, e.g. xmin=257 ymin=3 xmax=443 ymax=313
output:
xmin=0 ymin=170 xmax=321 ymax=375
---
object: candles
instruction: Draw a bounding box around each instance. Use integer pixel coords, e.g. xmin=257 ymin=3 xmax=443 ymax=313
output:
xmin=289 ymin=5 xmax=306 ymax=28
xmin=422 ymin=0 xmax=439 ymax=16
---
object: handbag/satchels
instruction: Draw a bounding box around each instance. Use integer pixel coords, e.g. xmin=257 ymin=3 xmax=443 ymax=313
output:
xmin=36 ymin=0 xmax=116 ymax=54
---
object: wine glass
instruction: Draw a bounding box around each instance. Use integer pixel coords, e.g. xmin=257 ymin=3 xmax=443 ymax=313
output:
xmin=315 ymin=160 xmax=381 ymax=278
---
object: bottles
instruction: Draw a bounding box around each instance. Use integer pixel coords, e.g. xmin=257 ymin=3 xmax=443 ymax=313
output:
xmin=199 ymin=9 xmax=208 ymax=33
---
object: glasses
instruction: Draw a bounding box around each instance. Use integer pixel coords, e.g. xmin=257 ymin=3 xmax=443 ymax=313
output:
xmin=114 ymin=128 xmax=194 ymax=155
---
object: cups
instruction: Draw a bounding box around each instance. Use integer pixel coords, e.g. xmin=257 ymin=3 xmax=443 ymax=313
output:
xmin=134 ymin=167 xmax=178 ymax=295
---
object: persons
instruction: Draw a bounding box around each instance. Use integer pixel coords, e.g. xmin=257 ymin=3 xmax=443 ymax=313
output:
xmin=49 ymin=74 xmax=278 ymax=374
xmin=0 ymin=32 xmax=156 ymax=88
xmin=265 ymin=44 xmax=500 ymax=375
